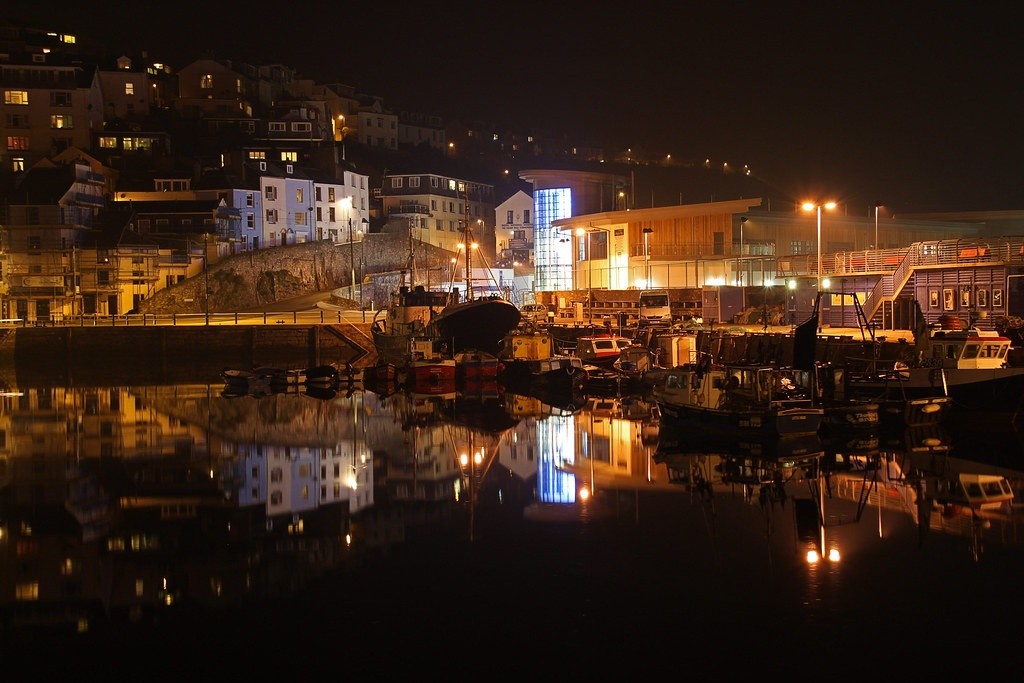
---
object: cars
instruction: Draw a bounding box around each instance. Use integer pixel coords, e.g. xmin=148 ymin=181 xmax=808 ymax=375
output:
xmin=520 ymin=303 xmax=548 ymax=323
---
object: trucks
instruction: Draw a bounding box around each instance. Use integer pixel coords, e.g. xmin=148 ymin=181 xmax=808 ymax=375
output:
xmin=701 ymin=285 xmax=744 ymax=324
xmin=639 ymin=290 xmax=672 ymax=334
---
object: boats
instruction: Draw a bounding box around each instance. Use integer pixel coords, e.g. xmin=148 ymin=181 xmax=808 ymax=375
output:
xmin=825 ymin=327 xmax=1024 ymax=389
xmin=655 ymin=360 xmax=771 ymax=428
xmin=774 ymin=360 xmax=824 ymax=437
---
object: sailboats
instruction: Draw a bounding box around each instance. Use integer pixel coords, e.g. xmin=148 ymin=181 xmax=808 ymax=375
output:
xmin=436 ymin=183 xmax=521 ymax=352
xmin=370 ymin=217 xmax=433 ymax=373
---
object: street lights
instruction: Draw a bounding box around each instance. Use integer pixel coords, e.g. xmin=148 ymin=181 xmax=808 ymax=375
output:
xmin=472 ymin=241 xmax=479 ymax=251
xmin=577 ymin=229 xmax=591 ymax=323
xmin=451 ymin=256 xmax=457 ymax=295
xmin=457 ymin=241 xmax=465 ymax=293
xmin=798 ymin=198 xmax=838 ymax=335
xmin=822 ymin=277 xmax=831 ymax=288
xmin=871 ymin=321 xmax=878 ymax=376
xmin=617 ymin=193 xmax=631 ymax=212
xmin=92 ymin=256 xmax=109 ymax=322
xmin=202 ymin=227 xmax=212 ymax=312
xmin=477 ymin=218 xmax=485 ymax=260
xmin=642 ymin=228 xmax=653 ymax=292
xmin=764 ymin=277 xmax=773 ymax=329
xmin=739 ymin=216 xmax=749 ymax=312
xmin=554 ymin=229 xmax=577 ymax=289
xmin=911 ymin=293 xmax=915 ymax=327
xmin=619 ymin=309 xmax=629 ymax=339
xmin=340 ymin=195 xmax=354 ymax=243
xmin=874 ymin=202 xmax=885 ymax=250
xmin=785 ymin=277 xmax=796 ymax=321
